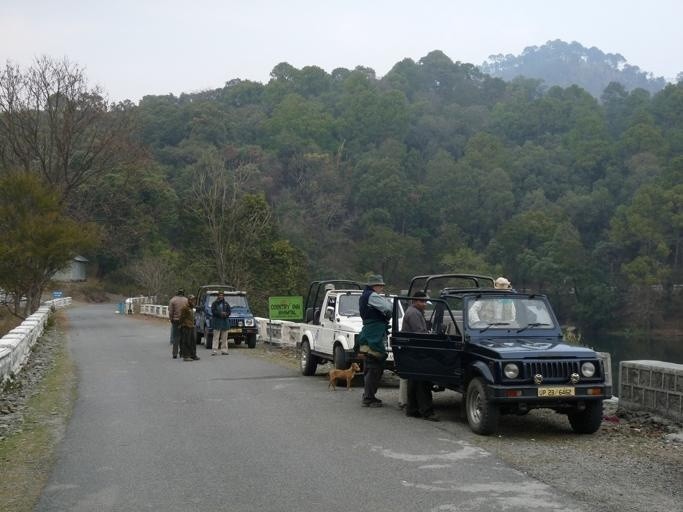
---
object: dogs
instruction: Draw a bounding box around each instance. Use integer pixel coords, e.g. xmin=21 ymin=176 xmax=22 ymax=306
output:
xmin=326 ymin=362 xmax=361 ymax=392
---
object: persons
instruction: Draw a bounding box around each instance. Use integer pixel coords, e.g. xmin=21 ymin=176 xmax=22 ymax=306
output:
xmin=353 ymin=274 xmax=397 ymax=408
xmin=210 ymin=289 xmax=231 ymax=357
xmin=168 ymin=288 xmax=200 ymax=362
xmin=402 ymin=292 xmax=429 ymax=332
xmin=467 ymin=277 xmax=517 ymax=329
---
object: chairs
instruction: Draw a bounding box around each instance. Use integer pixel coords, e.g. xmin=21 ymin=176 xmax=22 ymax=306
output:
xmin=305 ymin=306 xmax=320 ymax=324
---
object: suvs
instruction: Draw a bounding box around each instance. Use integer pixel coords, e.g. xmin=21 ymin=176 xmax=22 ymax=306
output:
xmin=190 ymin=283 xmax=258 ymax=349
xmin=0 ymin=290 xmax=15 ymax=304
xmin=389 ymin=273 xmax=611 ymax=437
xmin=297 ymin=276 xmax=405 ymax=386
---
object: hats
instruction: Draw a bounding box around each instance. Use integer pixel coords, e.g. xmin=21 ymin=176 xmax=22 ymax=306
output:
xmin=368 ymin=274 xmax=385 ymax=286
xmin=494 ymin=277 xmax=512 ymax=289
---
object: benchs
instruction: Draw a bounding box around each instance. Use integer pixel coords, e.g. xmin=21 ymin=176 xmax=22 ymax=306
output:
xmin=423 ymin=308 xmax=464 ymax=343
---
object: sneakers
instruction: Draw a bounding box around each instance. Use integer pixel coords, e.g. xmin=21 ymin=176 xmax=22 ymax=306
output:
xmin=362 ymin=398 xmax=382 ymax=407
xmin=171 ymin=351 xmax=228 ymax=362
